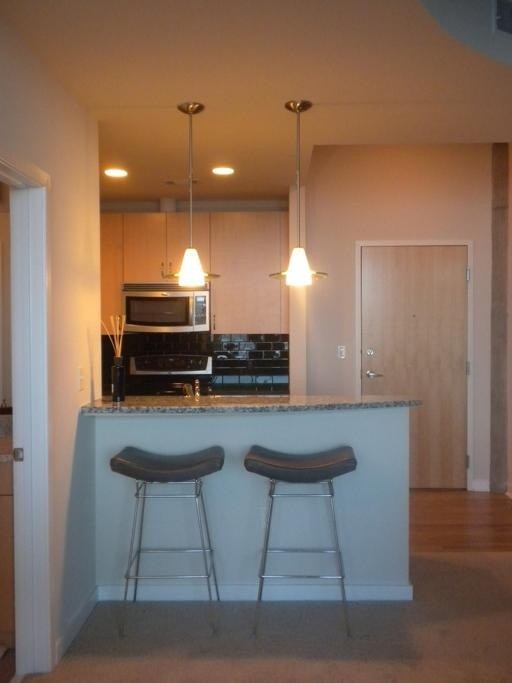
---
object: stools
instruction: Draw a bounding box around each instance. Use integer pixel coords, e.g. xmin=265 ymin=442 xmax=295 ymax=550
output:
xmin=109 ymin=447 xmax=227 ymax=639
xmin=243 ymin=445 xmax=358 ymax=638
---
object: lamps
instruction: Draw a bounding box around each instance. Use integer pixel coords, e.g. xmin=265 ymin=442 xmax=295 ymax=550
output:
xmin=269 ymin=98 xmax=329 ymax=288
xmin=162 ymin=101 xmax=220 ymax=289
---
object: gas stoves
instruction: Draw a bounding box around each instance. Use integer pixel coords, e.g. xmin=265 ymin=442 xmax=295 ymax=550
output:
xmin=128 ymin=353 xmax=212 ymax=397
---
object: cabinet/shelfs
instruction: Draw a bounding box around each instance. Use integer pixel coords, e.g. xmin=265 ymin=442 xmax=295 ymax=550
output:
xmin=123 ymin=212 xmax=211 ymax=285
xmin=209 ymin=212 xmax=289 ymax=334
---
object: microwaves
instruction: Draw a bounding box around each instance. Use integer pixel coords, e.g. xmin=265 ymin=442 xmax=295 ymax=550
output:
xmin=120 ymin=290 xmax=210 ymax=334
